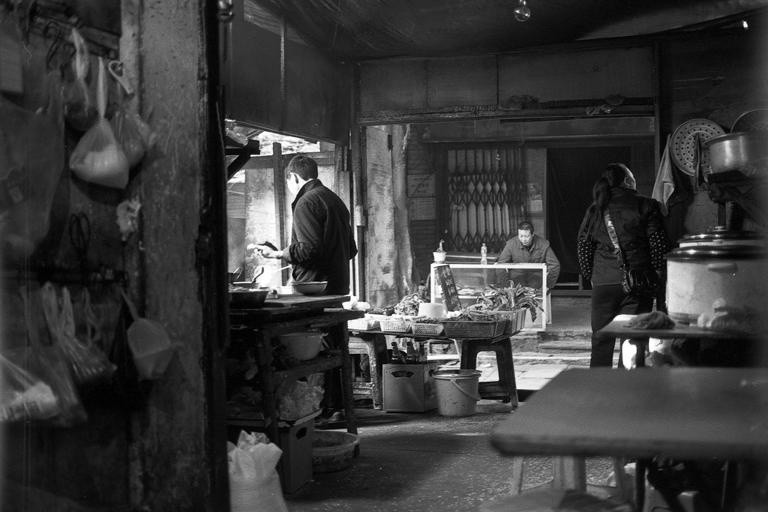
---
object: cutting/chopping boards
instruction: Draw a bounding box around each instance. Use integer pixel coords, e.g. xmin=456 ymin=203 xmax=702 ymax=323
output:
xmin=265 ymin=293 xmax=351 ymax=307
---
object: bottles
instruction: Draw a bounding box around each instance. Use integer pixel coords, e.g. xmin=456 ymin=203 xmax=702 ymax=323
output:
xmin=390 ymin=341 xmax=427 ymax=377
xmin=481 ymin=243 xmax=487 ymax=265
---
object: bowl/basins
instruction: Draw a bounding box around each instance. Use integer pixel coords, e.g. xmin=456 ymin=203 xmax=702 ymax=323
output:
xmin=228 ymin=273 xmax=240 ymax=283
xmin=278 ymin=331 xmax=327 ymax=362
xmin=703 ymin=131 xmax=767 ymax=173
xmin=226 ymin=288 xmax=270 ymax=308
xmin=292 ymin=281 xmax=328 ymax=295
xmin=311 ymin=430 xmax=360 ymax=471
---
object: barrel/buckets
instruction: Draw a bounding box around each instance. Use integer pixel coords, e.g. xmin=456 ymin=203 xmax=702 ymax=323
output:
xmin=431 ymin=368 xmax=482 ymax=417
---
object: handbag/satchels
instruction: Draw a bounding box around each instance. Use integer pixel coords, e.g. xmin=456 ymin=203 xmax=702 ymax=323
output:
xmin=621 ymin=258 xmax=662 ymax=297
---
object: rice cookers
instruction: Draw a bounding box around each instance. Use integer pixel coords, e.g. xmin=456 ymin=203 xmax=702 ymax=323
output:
xmin=665 ymin=226 xmax=768 ymax=328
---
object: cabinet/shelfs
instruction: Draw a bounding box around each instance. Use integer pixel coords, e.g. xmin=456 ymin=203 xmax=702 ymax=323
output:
xmin=226 ymin=310 xmax=364 ymax=456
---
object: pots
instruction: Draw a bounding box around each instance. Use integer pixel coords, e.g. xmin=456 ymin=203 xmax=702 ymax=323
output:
xmin=234 ymin=266 xmax=264 ymax=288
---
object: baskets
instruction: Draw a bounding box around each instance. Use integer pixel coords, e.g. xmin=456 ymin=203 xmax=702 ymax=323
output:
xmin=352 ymin=308 xmax=525 ymax=337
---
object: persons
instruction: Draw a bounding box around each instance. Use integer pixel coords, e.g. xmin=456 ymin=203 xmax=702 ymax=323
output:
xmin=253 ymin=154 xmax=359 ymax=429
xmin=497 ymin=221 xmax=561 ymax=295
xmin=576 ymin=164 xmax=671 ymax=368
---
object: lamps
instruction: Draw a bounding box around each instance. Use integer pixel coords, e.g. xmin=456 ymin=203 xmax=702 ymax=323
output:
xmin=513 ymin=0 xmax=531 ymax=22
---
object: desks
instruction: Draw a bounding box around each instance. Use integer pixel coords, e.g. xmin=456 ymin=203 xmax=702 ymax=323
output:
xmin=492 ymin=367 xmax=768 ymax=512
xmin=348 ymin=329 xmax=519 ymax=410
xmin=598 ymin=314 xmax=742 ymax=369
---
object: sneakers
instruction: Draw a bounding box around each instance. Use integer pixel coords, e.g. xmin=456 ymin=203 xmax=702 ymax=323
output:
xmin=327 ymin=409 xmax=345 ymax=422
